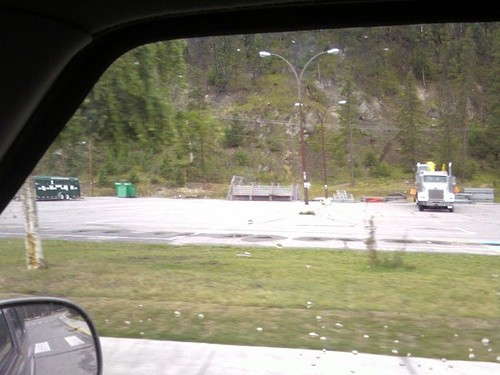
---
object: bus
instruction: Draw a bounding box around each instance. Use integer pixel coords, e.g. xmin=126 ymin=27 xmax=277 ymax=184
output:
xmin=15 ymin=176 xmax=80 ymax=201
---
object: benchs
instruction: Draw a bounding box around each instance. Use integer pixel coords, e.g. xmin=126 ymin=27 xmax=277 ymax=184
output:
xmin=230 ymin=184 xmax=295 ymax=201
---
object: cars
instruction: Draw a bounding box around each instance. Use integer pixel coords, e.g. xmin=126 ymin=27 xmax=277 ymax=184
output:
xmin=0 ymin=307 xmax=35 ymax=375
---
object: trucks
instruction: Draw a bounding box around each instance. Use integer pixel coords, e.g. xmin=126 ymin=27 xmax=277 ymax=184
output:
xmin=414 ymin=163 xmax=454 ymax=212
